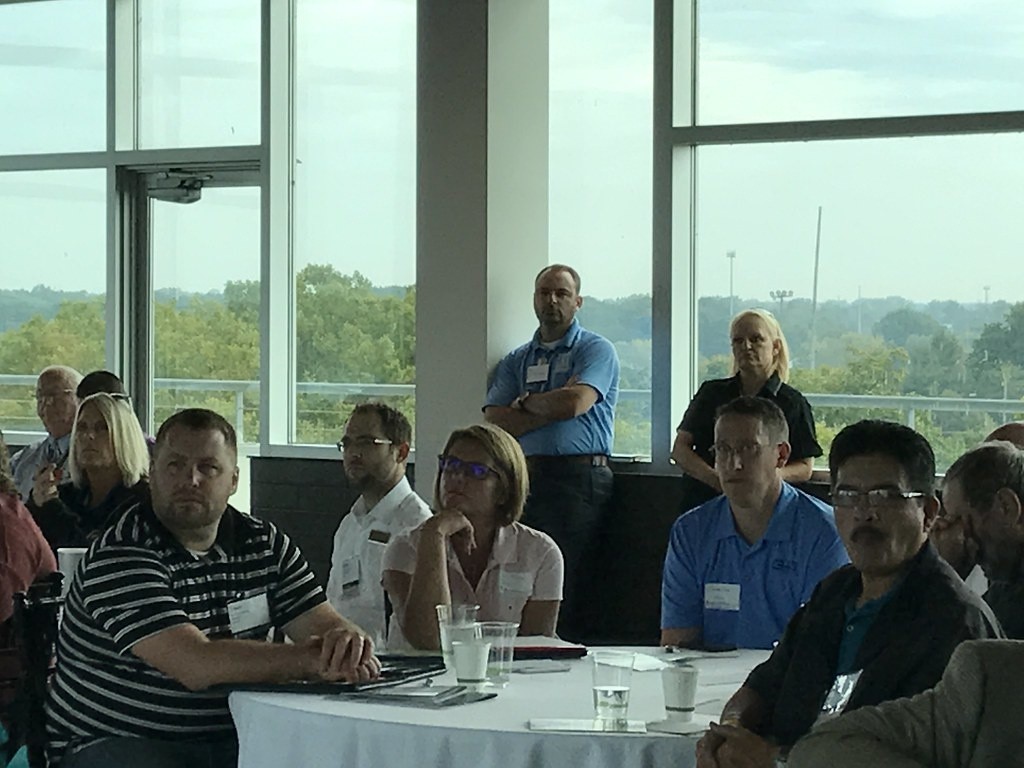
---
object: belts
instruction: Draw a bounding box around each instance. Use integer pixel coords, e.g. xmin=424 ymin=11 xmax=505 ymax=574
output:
xmin=529 ymin=454 xmax=608 ymax=467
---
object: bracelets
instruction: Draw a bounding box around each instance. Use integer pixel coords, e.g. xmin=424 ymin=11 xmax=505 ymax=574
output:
xmin=419 ymin=526 xmax=445 ymax=538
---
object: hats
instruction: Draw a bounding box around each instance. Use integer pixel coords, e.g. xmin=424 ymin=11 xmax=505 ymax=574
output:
xmin=76 ymin=370 xmax=129 ymax=401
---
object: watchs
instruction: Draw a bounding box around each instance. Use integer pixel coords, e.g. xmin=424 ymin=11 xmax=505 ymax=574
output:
xmin=518 ymin=391 xmax=531 ymax=412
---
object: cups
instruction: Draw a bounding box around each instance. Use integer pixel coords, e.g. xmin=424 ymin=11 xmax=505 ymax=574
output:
xmin=590 ymin=650 xmax=635 ymax=719
xmin=57 ymin=547 xmax=88 ymax=596
xmin=436 ymin=604 xmax=480 ymax=671
xmin=661 ymin=663 xmax=698 ymax=722
xmin=472 ymin=620 xmax=520 ymax=682
xmin=452 ymin=640 xmax=490 ymax=688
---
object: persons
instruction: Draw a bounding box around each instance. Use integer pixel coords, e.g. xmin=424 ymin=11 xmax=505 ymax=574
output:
xmin=38 ymin=407 xmax=383 ymax=768
xmin=321 ymin=405 xmax=434 ymax=656
xmin=695 ymin=419 xmax=1023 ymax=768
xmin=661 ymin=397 xmax=853 ymax=655
xmin=381 ymin=423 xmax=565 ymax=657
xmin=0 ymin=370 xmax=150 ymax=738
xmin=479 ymin=264 xmax=619 ymax=646
xmin=663 ymin=312 xmax=822 ymax=561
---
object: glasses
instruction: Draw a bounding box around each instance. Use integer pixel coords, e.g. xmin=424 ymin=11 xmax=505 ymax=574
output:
xmin=336 ymin=437 xmax=396 ymax=453
xmin=709 ymin=441 xmax=784 ymax=459
xmin=436 ymin=453 xmax=502 ymax=480
xmin=828 ymin=487 xmax=929 ymax=507
xmin=31 ymin=388 xmax=73 ymax=406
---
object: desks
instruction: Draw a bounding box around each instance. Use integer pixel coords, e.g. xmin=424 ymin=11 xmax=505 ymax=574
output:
xmin=227 ymin=648 xmax=863 ymax=768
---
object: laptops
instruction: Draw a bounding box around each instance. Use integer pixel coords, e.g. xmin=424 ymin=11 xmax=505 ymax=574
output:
xmin=487 ymin=634 xmax=587 ymax=660
